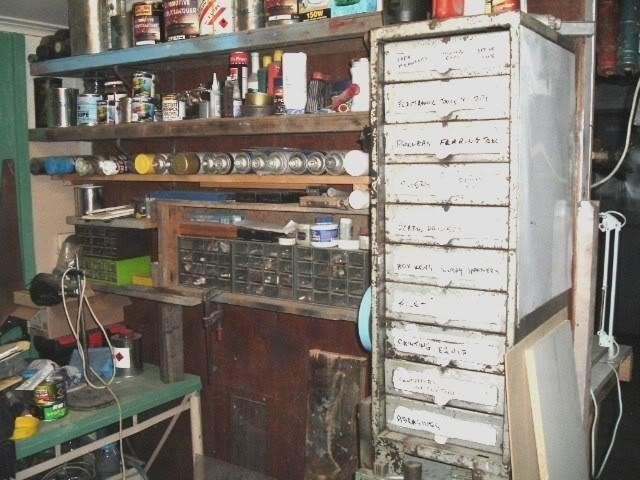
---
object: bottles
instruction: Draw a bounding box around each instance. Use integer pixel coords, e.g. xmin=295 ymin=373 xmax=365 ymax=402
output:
xmin=96 ymin=442 xmax=120 ymax=480
xmin=295 ymin=216 xmax=370 ymax=248
xmin=110 ymin=328 xmax=143 ymax=379
xmin=77 ymin=51 xmax=370 ymax=126
xmin=30 ymin=147 xmax=372 ymax=178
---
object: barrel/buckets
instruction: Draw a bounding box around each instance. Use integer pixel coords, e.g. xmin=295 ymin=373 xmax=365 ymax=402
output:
xmin=44 ymin=87 xmax=78 ymax=128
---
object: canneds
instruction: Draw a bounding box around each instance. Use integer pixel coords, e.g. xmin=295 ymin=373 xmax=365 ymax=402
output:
xmin=76 ymin=71 xmax=186 ymax=126
xmin=33 ymin=373 xmax=68 ymax=422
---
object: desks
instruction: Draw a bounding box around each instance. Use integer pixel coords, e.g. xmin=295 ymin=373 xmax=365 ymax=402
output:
xmin=13 ymin=364 xmax=204 ymax=479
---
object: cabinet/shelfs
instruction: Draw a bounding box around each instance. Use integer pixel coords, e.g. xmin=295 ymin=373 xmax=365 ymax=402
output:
xmin=29 ymin=12 xmax=375 ymax=474
xmin=375 ymin=14 xmax=589 ymax=457
